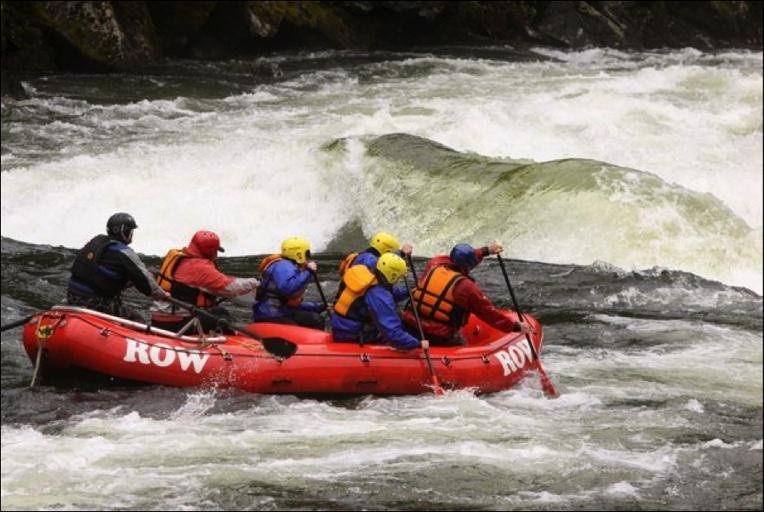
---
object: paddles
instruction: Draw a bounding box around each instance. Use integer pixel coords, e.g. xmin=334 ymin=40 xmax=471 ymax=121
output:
xmin=496 ymin=253 xmax=559 ymax=399
xmin=164 ymin=294 xmax=298 ymax=359
xmin=402 ymin=275 xmax=445 ymax=397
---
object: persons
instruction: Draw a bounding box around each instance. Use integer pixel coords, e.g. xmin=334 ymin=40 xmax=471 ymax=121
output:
xmin=331 ymin=231 xmax=413 ymax=305
xmin=400 ymin=238 xmax=530 ymax=347
xmin=63 ymin=212 xmax=171 ymax=326
xmin=250 ymin=236 xmax=331 ymax=330
xmin=148 ymin=228 xmax=263 ymax=335
xmin=327 ymin=251 xmax=430 ymax=352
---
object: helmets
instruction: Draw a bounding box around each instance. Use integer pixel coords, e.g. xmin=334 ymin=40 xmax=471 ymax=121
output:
xmin=106 ymin=212 xmax=139 ymax=243
xmin=449 ymin=243 xmax=478 ymax=268
xmin=190 ymin=230 xmax=225 ymax=261
xmin=281 ymin=236 xmax=311 ymax=265
xmin=370 ymin=232 xmax=409 ymax=286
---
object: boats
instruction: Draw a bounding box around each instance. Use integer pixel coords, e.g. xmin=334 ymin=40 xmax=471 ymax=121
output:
xmin=21 ymin=303 xmax=545 ymax=400
